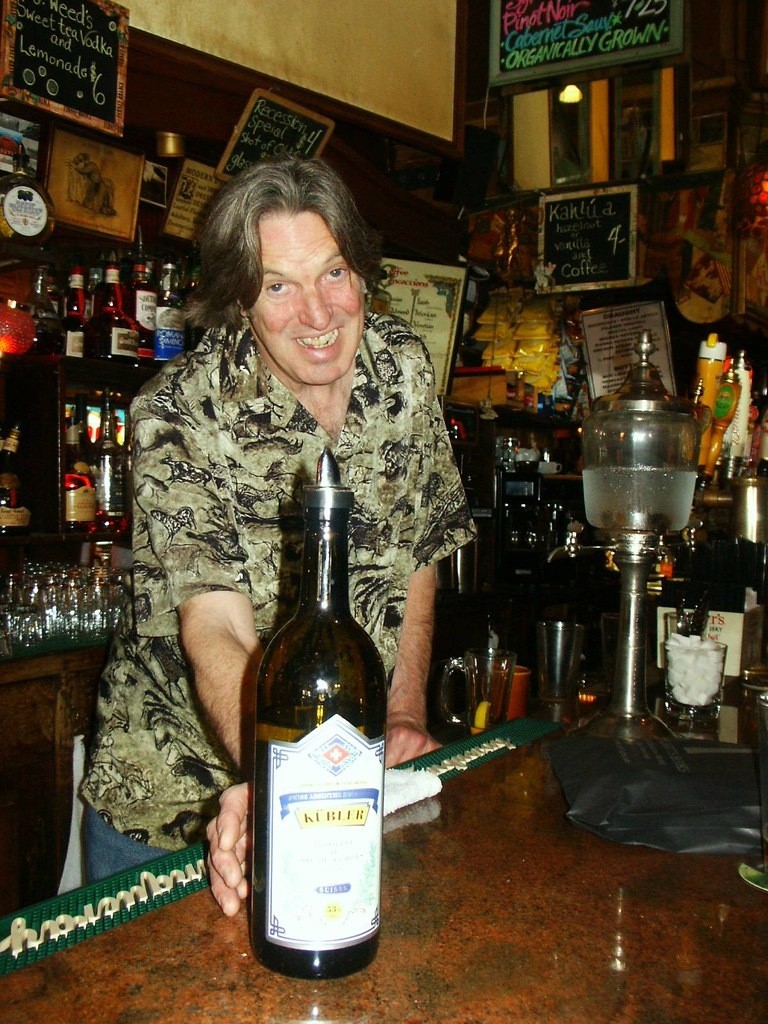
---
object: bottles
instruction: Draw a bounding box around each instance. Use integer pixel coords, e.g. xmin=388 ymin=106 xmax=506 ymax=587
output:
xmin=24 ymin=239 xmax=211 ymax=369
xmin=247 ymin=447 xmax=388 ymax=982
xmin=64 ymin=386 xmax=133 ymax=535
xmin=0 ymin=426 xmax=33 ymax=536
xmin=0 ymin=144 xmax=55 ymax=249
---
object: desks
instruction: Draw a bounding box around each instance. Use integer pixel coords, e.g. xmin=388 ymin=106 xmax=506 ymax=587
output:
xmin=0 ymin=697 xmax=768 ymax=1023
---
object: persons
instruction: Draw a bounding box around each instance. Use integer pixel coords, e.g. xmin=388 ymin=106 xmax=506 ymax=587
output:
xmin=78 ymin=160 xmax=479 ymax=915
xmin=492 ymin=207 xmax=518 ymax=272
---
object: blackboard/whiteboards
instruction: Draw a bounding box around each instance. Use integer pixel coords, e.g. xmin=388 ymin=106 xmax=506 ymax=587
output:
xmin=538 ymin=183 xmax=637 ymax=292
xmin=489 ymin=0 xmax=685 ymax=90
xmin=0 ymin=0 xmax=130 ymax=137
xmin=214 ymin=88 xmax=335 ymax=187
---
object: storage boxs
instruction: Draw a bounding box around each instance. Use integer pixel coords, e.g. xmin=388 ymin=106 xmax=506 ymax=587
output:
xmin=656 ymin=605 xmax=763 ymax=676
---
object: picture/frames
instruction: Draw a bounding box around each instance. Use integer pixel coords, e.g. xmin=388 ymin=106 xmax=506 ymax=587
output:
xmin=34 ymin=117 xmax=149 ymax=245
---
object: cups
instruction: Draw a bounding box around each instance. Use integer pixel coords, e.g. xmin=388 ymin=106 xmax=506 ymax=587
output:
xmin=600 ymin=614 xmax=619 ymax=697
xmin=438 ymin=648 xmax=517 ymax=736
xmin=507 ymin=665 xmax=532 ymax=722
xmin=536 ymin=620 xmax=585 ymax=706
xmin=538 ymin=462 xmax=562 ymax=474
xmin=0 ymin=559 xmax=128 ymax=659
xmin=663 ymin=612 xmax=708 ymax=639
xmin=741 ymin=606 xmax=768 ymax=691
xmin=665 ymin=639 xmax=727 ymax=734
